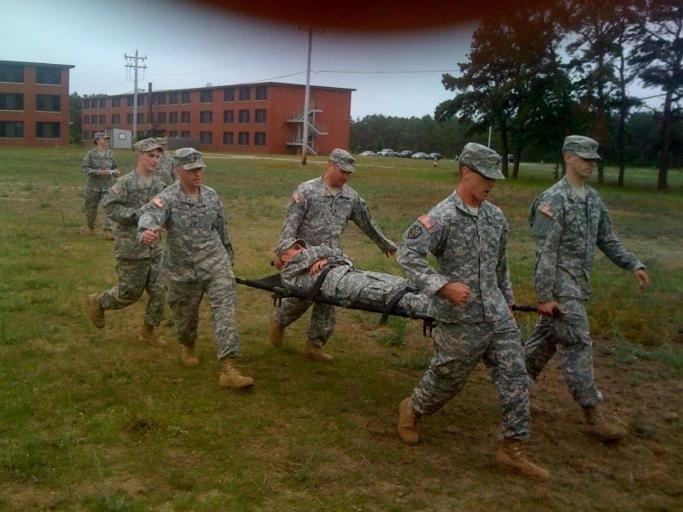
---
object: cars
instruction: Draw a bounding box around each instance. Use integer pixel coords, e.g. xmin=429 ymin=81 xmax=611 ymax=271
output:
xmin=358 ymin=148 xmax=443 ymax=161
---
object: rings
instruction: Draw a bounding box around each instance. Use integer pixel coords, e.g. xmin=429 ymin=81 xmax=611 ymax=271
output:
xmin=149 ymin=240 xmax=152 ymax=243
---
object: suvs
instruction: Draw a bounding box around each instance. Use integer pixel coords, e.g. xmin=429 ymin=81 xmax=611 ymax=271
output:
xmin=499 ymin=153 xmax=514 ymax=164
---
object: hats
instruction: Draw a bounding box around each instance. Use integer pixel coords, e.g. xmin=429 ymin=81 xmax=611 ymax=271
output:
xmin=458 ymin=143 xmax=506 ymax=180
xmin=92 ymin=132 xmax=206 ymax=171
xmin=328 ymin=148 xmax=356 ymax=174
xmin=563 ymin=136 xmax=603 ymax=159
xmin=275 ymin=237 xmax=306 ymax=257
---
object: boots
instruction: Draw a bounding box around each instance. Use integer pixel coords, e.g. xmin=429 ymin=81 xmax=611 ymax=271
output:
xmin=581 ymin=406 xmax=627 ymax=439
xmin=496 ymin=437 xmax=550 ymax=481
xmin=301 ymin=340 xmax=333 ymax=362
xmin=218 ymin=354 xmax=254 ymax=387
xmin=87 ymin=294 xmax=105 ymax=328
xmin=271 ymin=311 xmax=283 ymax=347
xmin=181 ymin=342 xmax=199 ymax=367
xmin=103 ymin=230 xmax=114 ymax=241
xmin=138 ymin=323 xmax=167 ymax=348
xmin=397 ymin=398 xmax=420 ymax=444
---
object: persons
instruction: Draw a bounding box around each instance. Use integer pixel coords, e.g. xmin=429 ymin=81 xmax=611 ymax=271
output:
xmin=524 ymin=135 xmax=649 ymax=439
xmin=88 ymin=138 xmax=168 ymax=347
xmin=80 ymin=133 xmax=120 ymax=240
xmin=270 ymin=148 xmax=397 ymax=360
xmin=154 ymin=137 xmax=178 ymax=188
xmin=137 ymin=148 xmax=254 ymax=389
xmin=397 ymin=142 xmax=550 ymax=481
xmin=276 ymin=237 xmax=434 ymax=317
